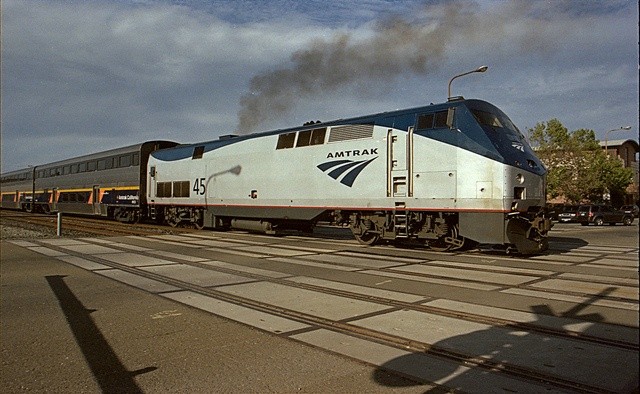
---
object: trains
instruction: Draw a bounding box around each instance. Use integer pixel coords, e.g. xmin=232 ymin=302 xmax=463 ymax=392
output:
xmin=1 ymin=98 xmax=554 ymax=260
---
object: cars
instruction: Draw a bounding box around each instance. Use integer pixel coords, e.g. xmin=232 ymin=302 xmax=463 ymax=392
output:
xmin=558 ymin=206 xmax=579 ymax=222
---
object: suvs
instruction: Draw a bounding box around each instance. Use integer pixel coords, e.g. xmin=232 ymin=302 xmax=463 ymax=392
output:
xmin=577 ymin=204 xmax=634 ymax=226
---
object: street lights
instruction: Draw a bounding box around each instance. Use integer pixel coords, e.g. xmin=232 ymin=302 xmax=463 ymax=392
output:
xmin=448 ymin=64 xmax=487 ymax=100
xmin=604 ymin=124 xmax=632 ymax=158
